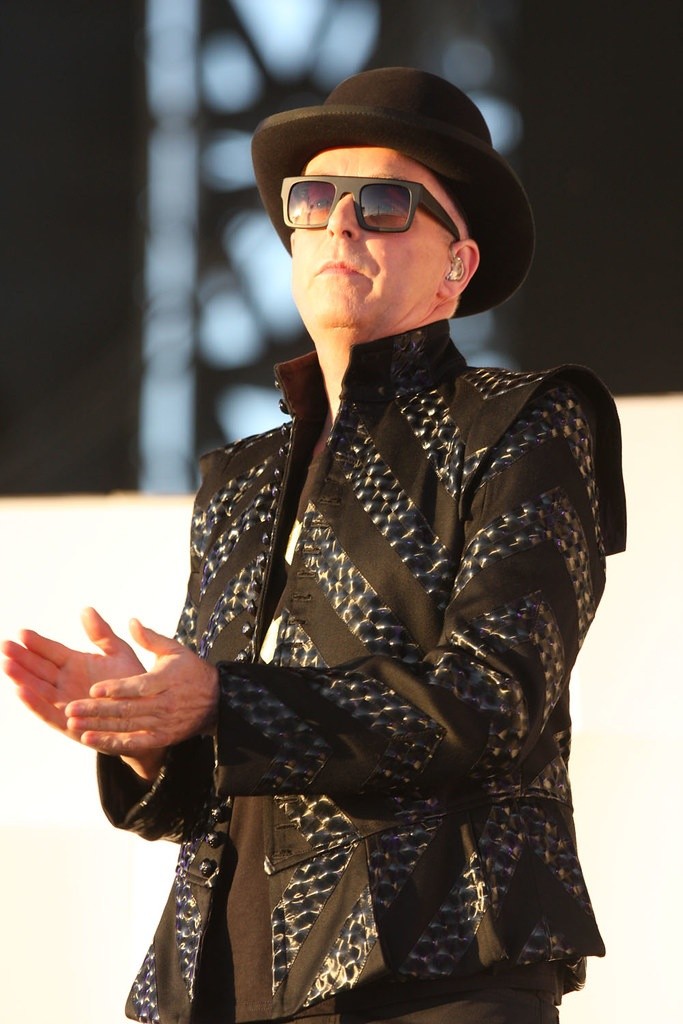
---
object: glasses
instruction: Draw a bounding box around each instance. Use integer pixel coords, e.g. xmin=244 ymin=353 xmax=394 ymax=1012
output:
xmin=280 ymin=174 xmax=462 ymax=241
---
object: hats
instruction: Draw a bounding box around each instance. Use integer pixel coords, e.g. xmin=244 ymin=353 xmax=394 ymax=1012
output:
xmin=251 ymin=68 xmax=535 ymax=319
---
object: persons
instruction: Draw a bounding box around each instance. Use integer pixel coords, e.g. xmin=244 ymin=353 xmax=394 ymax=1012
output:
xmin=2 ymin=66 xmax=629 ymax=1024
